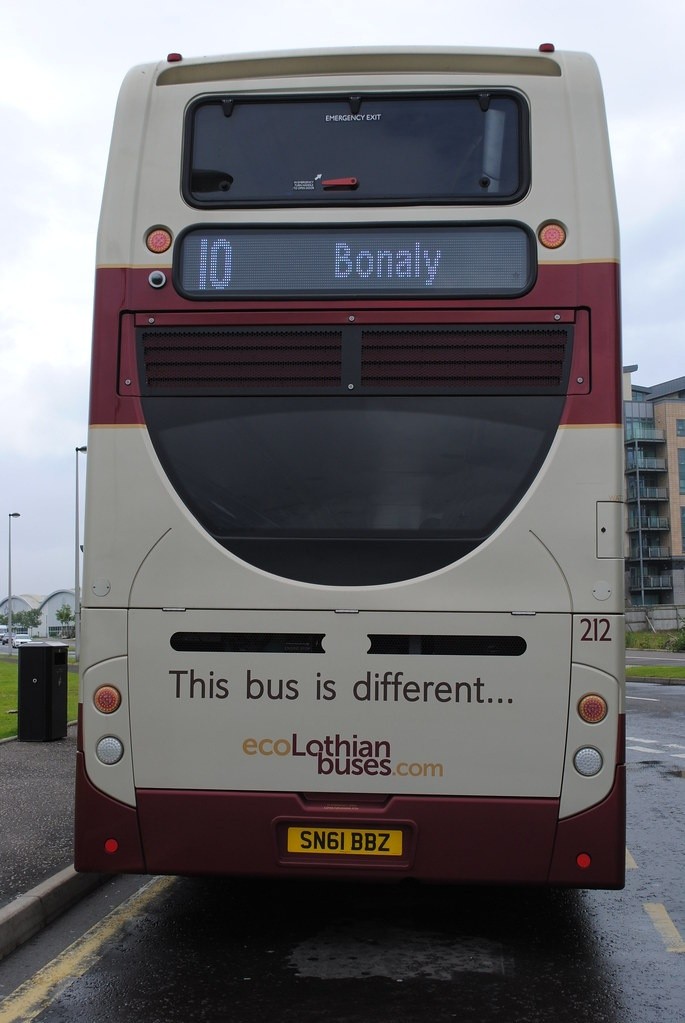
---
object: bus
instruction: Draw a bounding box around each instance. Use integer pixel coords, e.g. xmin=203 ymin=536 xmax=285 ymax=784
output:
xmin=69 ymin=42 xmax=635 ymax=896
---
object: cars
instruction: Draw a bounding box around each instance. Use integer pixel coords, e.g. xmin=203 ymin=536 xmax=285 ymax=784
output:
xmin=0 ymin=632 xmax=32 ymax=649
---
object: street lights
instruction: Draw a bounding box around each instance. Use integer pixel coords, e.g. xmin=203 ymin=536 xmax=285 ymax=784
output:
xmin=74 ymin=444 xmax=88 ymax=664
xmin=8 ymin=512 xmax=22 ymax=658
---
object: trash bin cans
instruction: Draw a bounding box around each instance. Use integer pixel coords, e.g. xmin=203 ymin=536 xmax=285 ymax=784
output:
xmin=14 ymin=640 xmax=69 ymax=741
